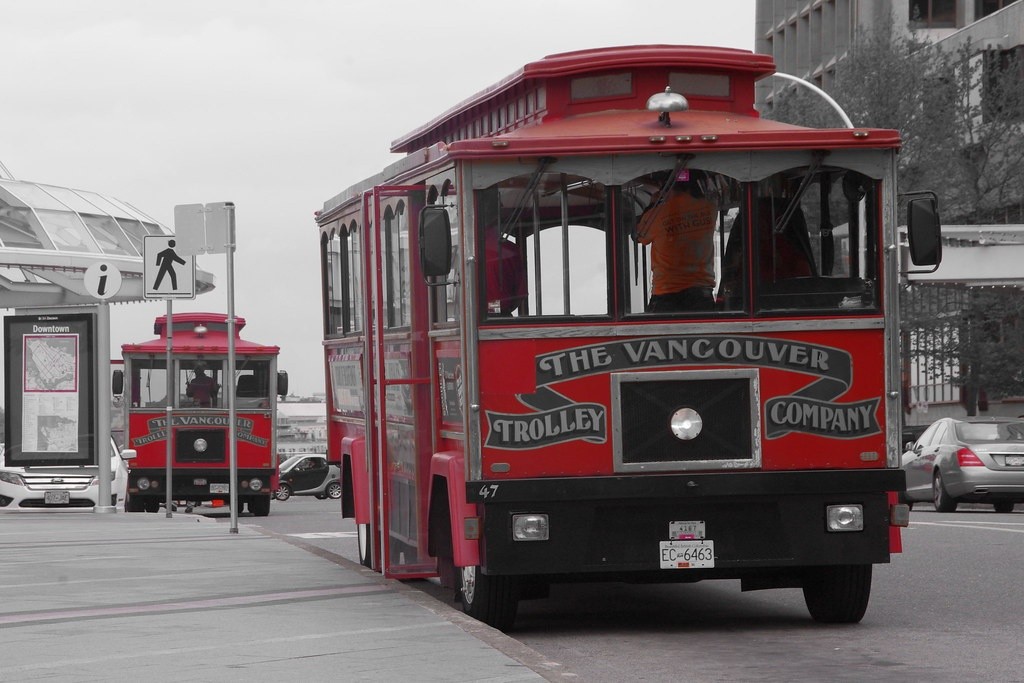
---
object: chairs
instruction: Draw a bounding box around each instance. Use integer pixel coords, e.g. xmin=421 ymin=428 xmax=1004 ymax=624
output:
xmin=236 ymin=375 xmax=259 ymax=398
xmin=725 ymin=193 xmax=817 ymax=310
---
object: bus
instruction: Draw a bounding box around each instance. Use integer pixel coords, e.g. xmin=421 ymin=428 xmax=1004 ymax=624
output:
xmin=109 ymin=310 xmax=291 ymax=517
xmin=311 ymin=43 xmax=943 ymax=639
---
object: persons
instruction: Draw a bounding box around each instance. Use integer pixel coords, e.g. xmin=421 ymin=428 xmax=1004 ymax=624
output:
xmin=185 ymin=364 xmax=219 ymax=407
xmin=310 ymin=459 xmax=319 ymax=469
xmin=631 ymin=169 xmax=720 ymax=315
xmin=483 ymin=193 xmax=530 ymax=319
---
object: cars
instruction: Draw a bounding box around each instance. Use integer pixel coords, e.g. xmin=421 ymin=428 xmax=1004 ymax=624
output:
xmin=900 ymin=415 xmax=1024 ymax=522
xmin=0 ymin=431 xmax=140 ymax=514
xmin=274 ymin=453 xmax=343 ymax=504
